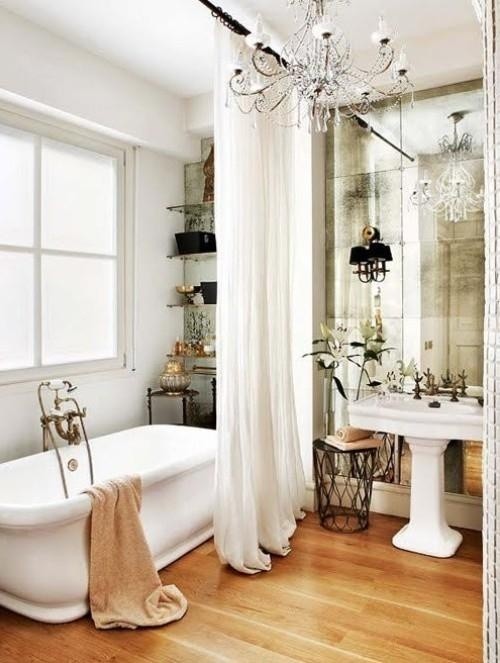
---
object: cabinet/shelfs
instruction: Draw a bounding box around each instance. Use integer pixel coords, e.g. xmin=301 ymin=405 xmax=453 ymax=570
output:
xmin=167 ymin=199 xmax=214 ymax=309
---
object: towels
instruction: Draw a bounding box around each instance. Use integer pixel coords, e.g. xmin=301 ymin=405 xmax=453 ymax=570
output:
xmin=80 ymin=474 xmax=189 ymax=630
xmin=324 ymin=423 xmax=377 ymax=451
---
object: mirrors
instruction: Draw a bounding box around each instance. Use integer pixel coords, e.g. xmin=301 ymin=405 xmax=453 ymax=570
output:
xmin=319 ymin=79 xmax=484 ymax=506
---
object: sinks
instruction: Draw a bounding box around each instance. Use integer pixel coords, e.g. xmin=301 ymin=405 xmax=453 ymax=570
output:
xmin=347 ymin=392 xmax=486 ymax=442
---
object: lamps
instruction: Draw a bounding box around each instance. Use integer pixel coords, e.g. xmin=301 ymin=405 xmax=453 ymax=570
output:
xmin=396 ymin=111 xmax=485 ymax=224
xmin=223 ymin=1 xmax=416 ymax=137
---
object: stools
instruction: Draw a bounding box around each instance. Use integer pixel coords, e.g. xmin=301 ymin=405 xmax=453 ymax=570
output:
xmin=310 ymin=443 xmax=375 ymax=535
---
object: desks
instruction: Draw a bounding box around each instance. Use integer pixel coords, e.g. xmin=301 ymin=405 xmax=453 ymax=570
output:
xmin=142 ymin=385 xmax=200 ymax=425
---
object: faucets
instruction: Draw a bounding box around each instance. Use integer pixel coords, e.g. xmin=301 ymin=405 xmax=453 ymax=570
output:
xmin=423 ymin=374 xmax=437 ymax=395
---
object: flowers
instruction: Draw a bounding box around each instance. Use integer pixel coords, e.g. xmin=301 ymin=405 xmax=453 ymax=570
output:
xmin=349 ymin=311 xmax=397 ymax=417
xmin=302 ymin=318 xmax=351 ymax=435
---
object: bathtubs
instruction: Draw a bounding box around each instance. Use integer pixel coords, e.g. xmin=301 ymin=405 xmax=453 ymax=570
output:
xmin=0 ymin=425 xmax=217 ymax=624
